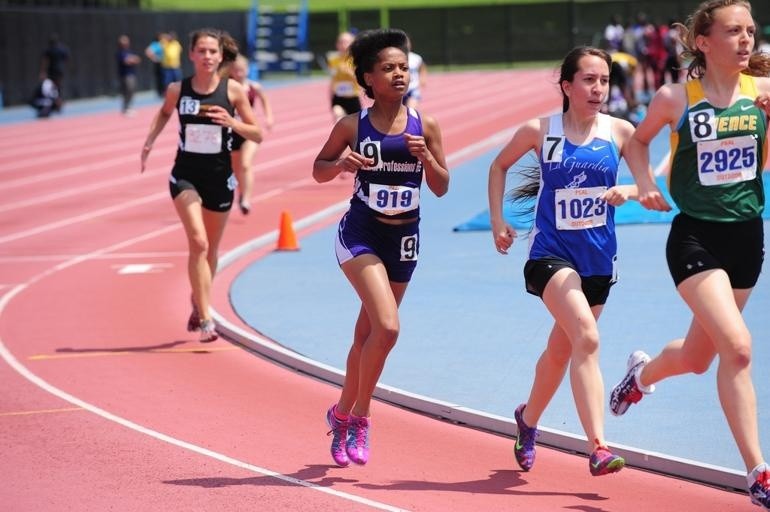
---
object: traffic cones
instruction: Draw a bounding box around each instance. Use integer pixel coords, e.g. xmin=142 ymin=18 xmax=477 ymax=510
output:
xmin=271 ymin=210 xmax=299 ymax=253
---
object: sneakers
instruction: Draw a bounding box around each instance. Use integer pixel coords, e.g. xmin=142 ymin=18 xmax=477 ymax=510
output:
xmin=345 ymin=399 xmax=371 ymax=465
xmin=187 ymin=294 xmax=205 ymax=333
xmin=746 ymin=463 xmax=769 ymax=512
xmin=326 ymin=402 xmax=353 ymax=468
xmin=589 ymin=437 xmax=625 ymax=476
xmin=611 ymin=350 xmax=654 ymax=417
xmin=514 ymin=404 xmax=540 ymax=472
xmin=198 ymin=318 xmax=218 ymax=342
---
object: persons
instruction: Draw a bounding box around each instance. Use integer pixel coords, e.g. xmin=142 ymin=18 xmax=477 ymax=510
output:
xmin=117 ymin=35 xmax=142 ymax=113
xmin=313 ymin=26 xmax=450 ymax=466
xmin=228 ymin=54 xmax=273 ymax=213
xmin=608 ymin=1 xmax=770 ymax=512
xmin=488 ymin=43 xmax=655 ymax=477
xmin=401 ymin=52 xmax=430 ymax=110
xmin=326 ymin=31 xmax=364 ymax=180
xmin=145 ymin=29 xmax=183 ymax=100
xmin=29 ymin=35 xmax=70 ymax=118
xmin=594 ymin=8 xmax=770 ymax=127
xmin=139 ymin=27 xmax=263 ymax=344
